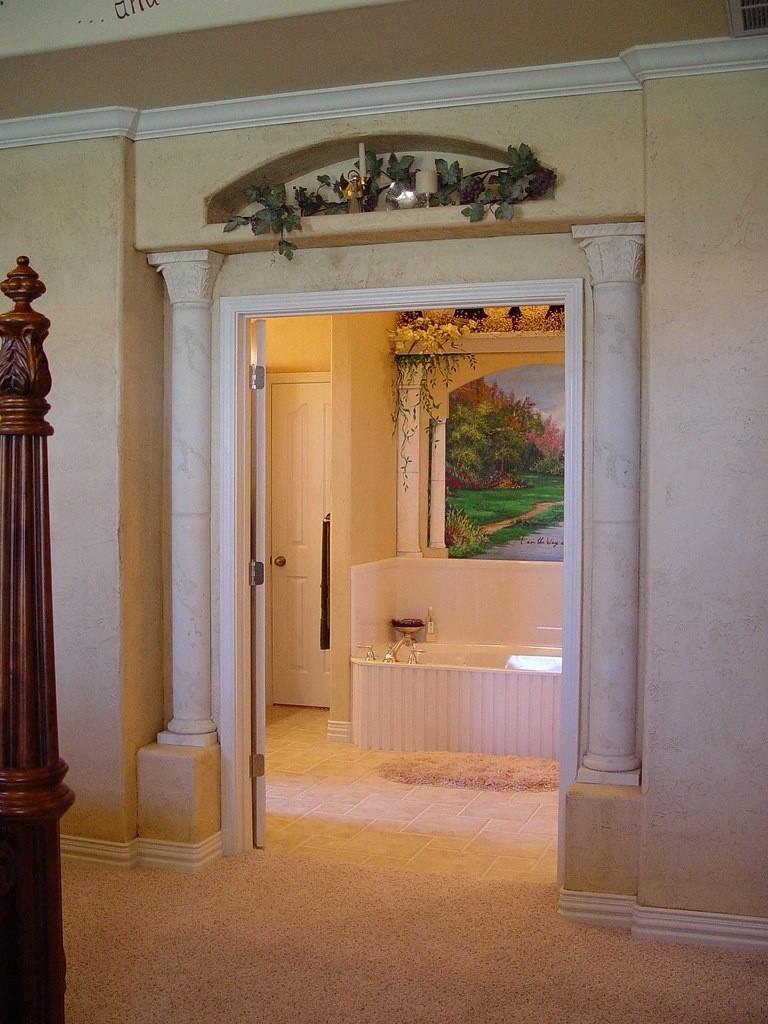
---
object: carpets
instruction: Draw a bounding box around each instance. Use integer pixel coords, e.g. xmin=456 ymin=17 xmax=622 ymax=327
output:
xmin=377 ymin=751 xmax=559 ymax=792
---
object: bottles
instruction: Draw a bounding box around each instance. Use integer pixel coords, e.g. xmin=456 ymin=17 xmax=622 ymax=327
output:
xmin=424 ymin=607 xmax=437 ymax=642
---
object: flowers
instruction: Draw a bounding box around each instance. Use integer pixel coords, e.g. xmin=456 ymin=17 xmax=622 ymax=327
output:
xmin=385 ymin=305 xmax=565 ymax=490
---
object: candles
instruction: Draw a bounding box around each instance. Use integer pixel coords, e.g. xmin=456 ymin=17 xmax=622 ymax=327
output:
xmin=416 ymin=171 xmax=438 ymax=193
xmin=359 ymin=143 xmax=366 ymax=178
